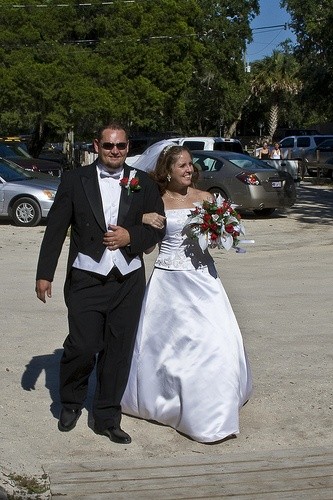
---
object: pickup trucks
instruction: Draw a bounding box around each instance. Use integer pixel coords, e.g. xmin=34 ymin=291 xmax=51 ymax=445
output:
xmin=124 ymin=136 xmax=299 ymax=181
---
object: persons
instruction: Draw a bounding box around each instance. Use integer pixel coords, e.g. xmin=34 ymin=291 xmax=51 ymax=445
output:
xmin=270 ymin=143 xmax=280 ymax=159
xmin=259 ymin=142 xmax=269 ymax=160
xmin=122 ymin=143 xmax=252 ymax=443
xmin=35 ymin=124 xmax=167 ymax=444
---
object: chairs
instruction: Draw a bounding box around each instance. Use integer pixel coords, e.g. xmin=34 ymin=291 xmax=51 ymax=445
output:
xmin=196 ymin=160 xmax=206 ymax=171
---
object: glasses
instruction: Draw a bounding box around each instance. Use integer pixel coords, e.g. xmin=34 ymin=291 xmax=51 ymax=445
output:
xmin=99 ymin=142 xmax=128 ymax=150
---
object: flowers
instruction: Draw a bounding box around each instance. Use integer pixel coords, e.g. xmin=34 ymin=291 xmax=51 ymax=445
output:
xmin=119 ymin=169 xmax=141 ymax=195
xmin=186 ymin=192 xmax=246 ymax=253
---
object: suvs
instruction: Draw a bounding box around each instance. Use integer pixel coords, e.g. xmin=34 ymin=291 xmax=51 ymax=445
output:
xmin=254 ymin=135 xmax=333 ymax=161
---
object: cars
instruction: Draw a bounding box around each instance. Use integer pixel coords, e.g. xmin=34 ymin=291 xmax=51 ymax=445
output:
xmin=303 ymin=139 xmax=333 ymax=178
xmin=0 ymin=142 xmax=64 ymax=228
xmin=189 ymin=150 xmax=296 ymax=216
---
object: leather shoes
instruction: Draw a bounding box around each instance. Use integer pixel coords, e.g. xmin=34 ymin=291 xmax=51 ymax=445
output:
xmin=93 ymin=426 xmax=131 ymax=443
xmin=58 ymin=406 xmax=82 ymax=432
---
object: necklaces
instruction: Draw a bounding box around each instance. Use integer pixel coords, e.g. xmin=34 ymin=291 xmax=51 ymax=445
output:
xmin=166 ymin=190 xmax=189 ymax=201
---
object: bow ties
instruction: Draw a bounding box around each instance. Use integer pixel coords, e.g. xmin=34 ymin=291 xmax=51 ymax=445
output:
xmin=100 ymin=172 xmax=120 ymax=180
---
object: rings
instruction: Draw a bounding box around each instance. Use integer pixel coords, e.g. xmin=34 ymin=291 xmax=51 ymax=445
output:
xmin=113 ymin=242 xmax=114 ymax=245
